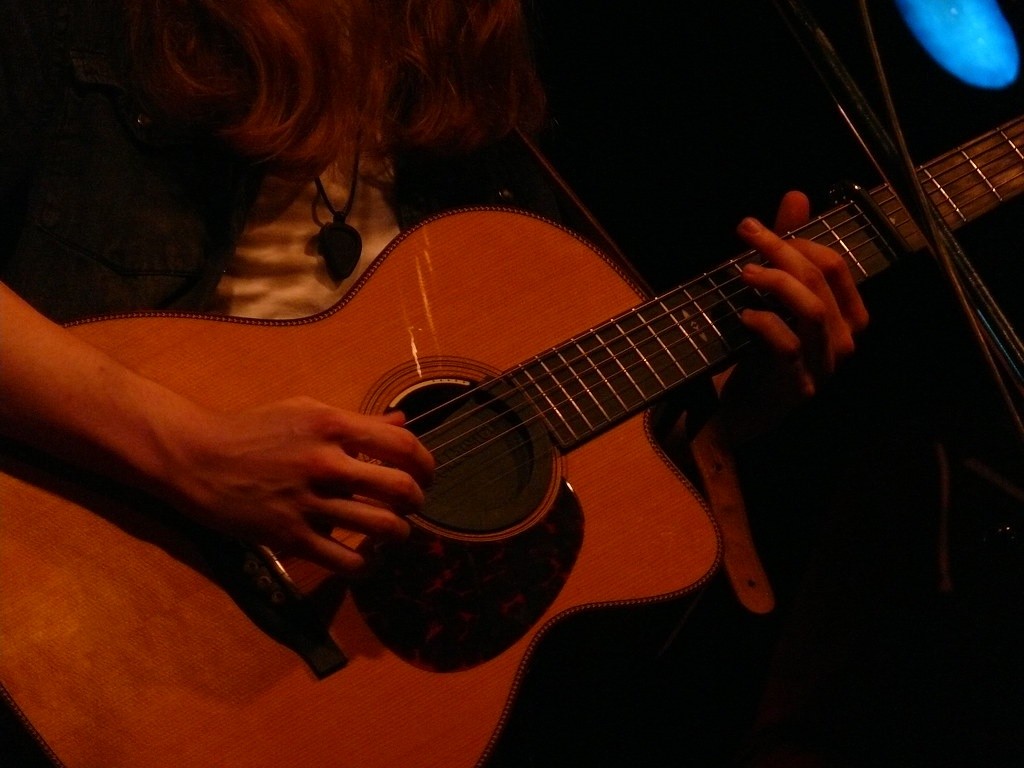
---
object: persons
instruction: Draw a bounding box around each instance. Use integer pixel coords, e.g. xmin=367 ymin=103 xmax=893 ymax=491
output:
xmin=0 ymin=0 xmax=870 ymax=768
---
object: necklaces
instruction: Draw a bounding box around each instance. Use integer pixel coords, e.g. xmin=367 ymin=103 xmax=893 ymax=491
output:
xmin=314 ymin=81 xmax=362 ymax=280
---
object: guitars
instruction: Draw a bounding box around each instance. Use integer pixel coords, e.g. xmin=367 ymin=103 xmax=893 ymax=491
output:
xmin=1 ymin=111 xmax=1022 ymax=768
